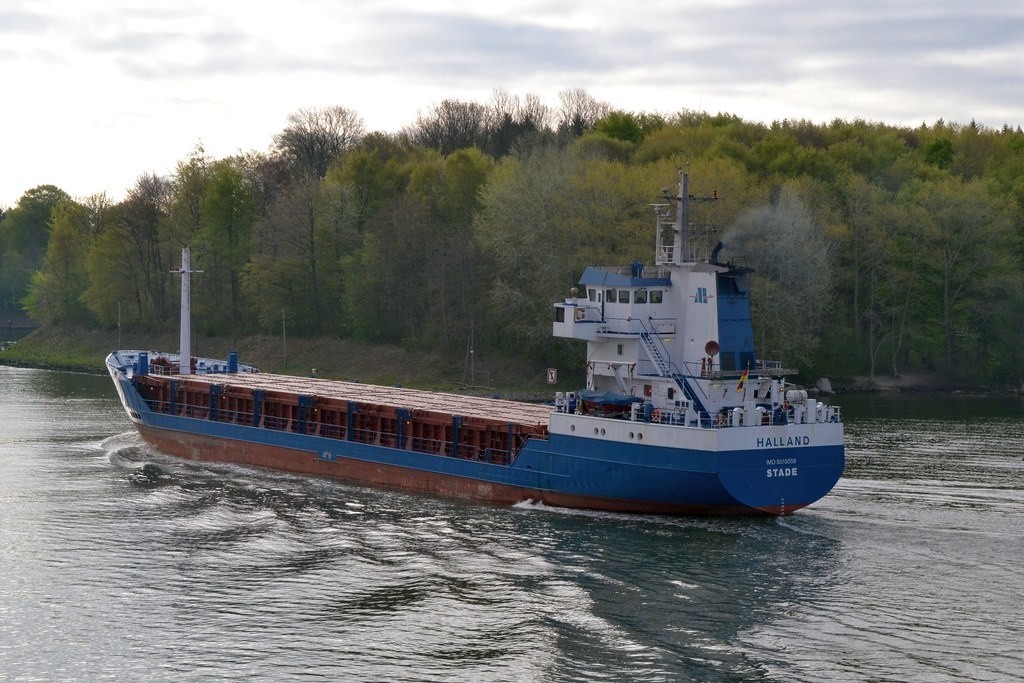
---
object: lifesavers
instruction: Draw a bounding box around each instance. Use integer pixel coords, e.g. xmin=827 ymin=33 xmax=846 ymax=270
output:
xmin=716 ymin=415 xmax=726 ymax=426
xmin=652 ymin=409 xmax=663 ymax=421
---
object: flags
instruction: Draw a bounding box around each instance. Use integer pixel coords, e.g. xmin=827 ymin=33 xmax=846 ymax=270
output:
xmin=736 ymin=369 xmax=747 ymax=392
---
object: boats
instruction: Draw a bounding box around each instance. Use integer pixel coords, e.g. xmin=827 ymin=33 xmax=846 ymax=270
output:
xmin=103 ymin=165 xmax=845 ymax=521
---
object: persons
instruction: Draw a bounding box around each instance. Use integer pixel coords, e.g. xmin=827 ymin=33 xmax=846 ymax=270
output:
xmin=565 ymin=393 xmax=578 ymax=415
